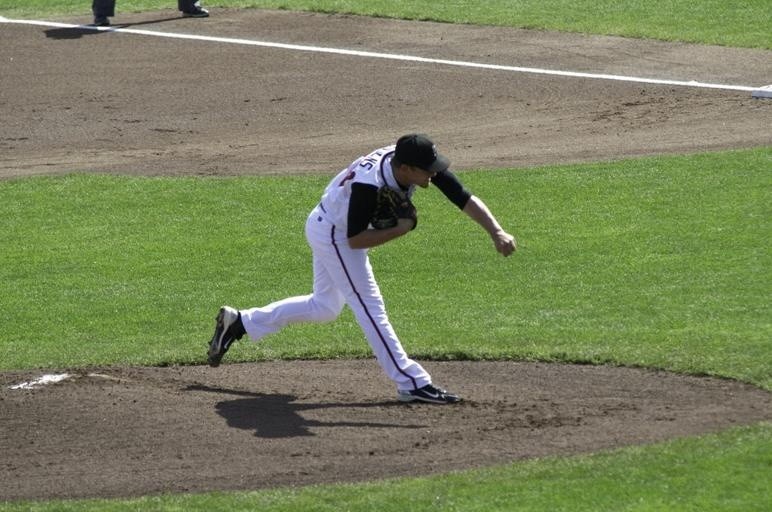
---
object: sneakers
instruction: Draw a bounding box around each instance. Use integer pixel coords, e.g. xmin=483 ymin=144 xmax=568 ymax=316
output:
xmin=397 ymin=384 xmax=463 ymax=405
xmin=182 ymin=7 xmax=210 ymax=17
xmin=93 ymin=15 xmax=109 ymax=25
xmin=207 ymin=305 xmax=247 ymax=367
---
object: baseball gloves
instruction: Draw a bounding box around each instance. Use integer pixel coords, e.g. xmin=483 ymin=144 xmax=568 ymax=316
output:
xmin=372 ymin=186 xmax=418 ymax=231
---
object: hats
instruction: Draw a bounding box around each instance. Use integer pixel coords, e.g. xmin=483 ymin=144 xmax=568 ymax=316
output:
xmin=396 ymin=133 xmax=450 ymax=173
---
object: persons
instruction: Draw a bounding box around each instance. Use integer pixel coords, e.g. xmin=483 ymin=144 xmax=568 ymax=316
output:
xmin=90 ymin=0 xmax=211 ymax=27
xmin=204 ymin=133 xmax=516 ymax=407
